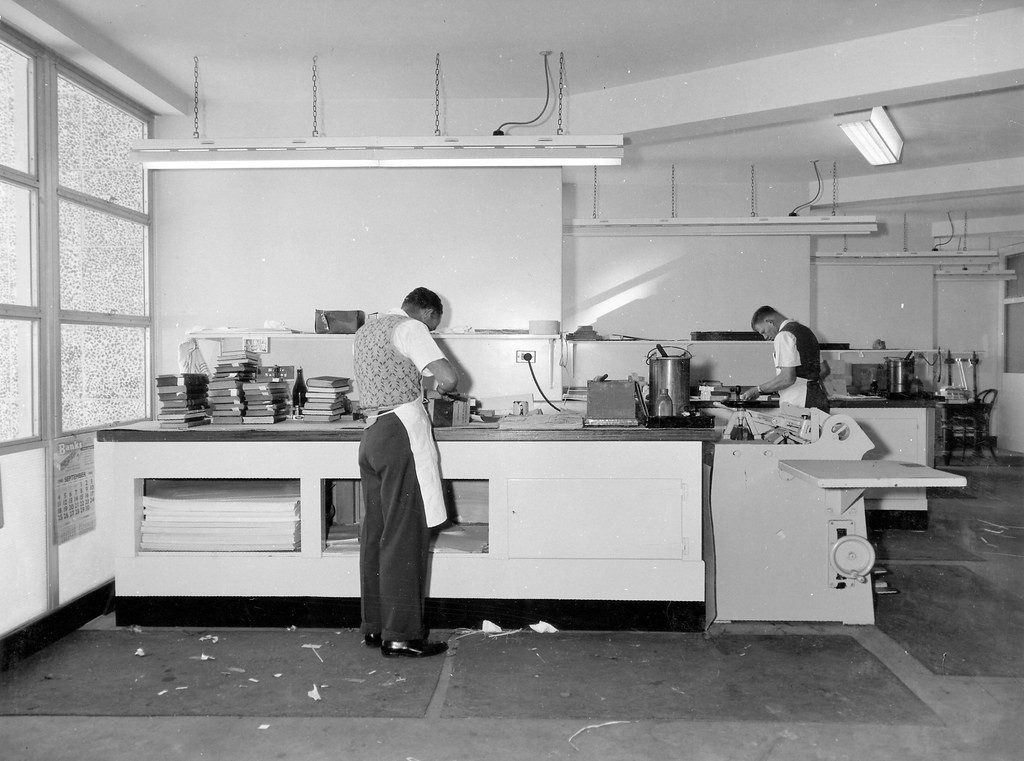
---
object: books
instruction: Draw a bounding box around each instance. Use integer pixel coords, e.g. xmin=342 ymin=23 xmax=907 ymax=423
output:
xmin=155 ymin=349 xmax=351 ymax=430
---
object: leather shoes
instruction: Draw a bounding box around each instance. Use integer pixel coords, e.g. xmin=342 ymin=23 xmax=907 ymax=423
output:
xmin=381 ymin=639 xmax=449 ymax=658
xmin=364 ymin=632 xmax=386 ymax=649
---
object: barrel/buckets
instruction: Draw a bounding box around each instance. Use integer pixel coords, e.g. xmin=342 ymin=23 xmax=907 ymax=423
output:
xmin=646 ymin=346 xmax=692 ymax=417
xmin=883 ymin=356 xmax=915 ymax=394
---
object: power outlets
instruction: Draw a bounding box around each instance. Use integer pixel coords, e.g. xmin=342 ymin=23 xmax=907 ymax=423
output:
xmin=517 ymin=350 xmax=536 ymax=363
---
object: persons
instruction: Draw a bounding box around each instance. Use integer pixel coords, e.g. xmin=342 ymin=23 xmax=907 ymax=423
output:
xmin=353 ymin=286 xmax=459 ymax=659
xmin=741 ymin=305 xmax=830 ymax=414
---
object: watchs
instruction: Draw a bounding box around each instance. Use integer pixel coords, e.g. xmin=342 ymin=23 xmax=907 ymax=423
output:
xmin=756 ymin=385 xmax=764 ymax=395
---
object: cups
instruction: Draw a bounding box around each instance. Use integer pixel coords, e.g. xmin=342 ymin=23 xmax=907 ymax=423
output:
xmin=513 ymin=401 xmax=529 ymax=416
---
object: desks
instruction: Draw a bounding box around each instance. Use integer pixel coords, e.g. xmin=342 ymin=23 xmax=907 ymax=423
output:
xmin=937 ymin=402 xmax=993 ymax=465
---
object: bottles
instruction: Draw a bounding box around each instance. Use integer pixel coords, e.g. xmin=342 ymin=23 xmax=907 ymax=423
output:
xmin=869 ymin=379 xmax=879 ymax=394
xmin=293 ymin=369 xmax=307 ymax=414
xmin=655 ymin=389 xmax=672 ymax=416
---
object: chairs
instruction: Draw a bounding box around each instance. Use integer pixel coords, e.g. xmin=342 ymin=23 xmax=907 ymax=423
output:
xmin=941 ymin=389 xmax=998 ymax=461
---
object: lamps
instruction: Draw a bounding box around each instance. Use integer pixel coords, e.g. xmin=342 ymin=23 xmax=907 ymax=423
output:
xmin=932 ymin=238 xmax=1019 ymax=282
xmin=562 ymin=161 xmax=878 ymax=236
xmin=811 ymin=211 xmax=1000 ymax=266
xmin=129 ymin=52 xmax=625 ymax=169
